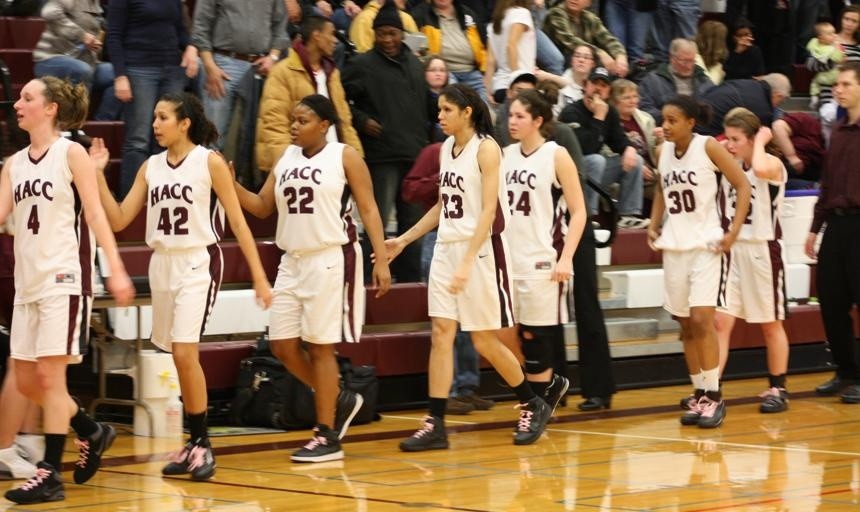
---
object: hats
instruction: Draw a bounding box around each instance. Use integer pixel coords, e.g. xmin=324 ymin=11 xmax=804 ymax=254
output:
xmin=590 ymin=65 xmax=611 ymax=84
xmin=374 ymin=0 xmax=404 ymax=32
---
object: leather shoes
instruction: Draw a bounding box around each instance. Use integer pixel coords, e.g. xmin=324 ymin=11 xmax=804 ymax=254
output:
xmin=817 ymin=377 xmax=860 ymax=403
xmin=579 ymin=396 xmax=610 ymax=411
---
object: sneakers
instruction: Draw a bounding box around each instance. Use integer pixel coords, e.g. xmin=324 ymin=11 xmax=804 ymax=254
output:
xmin=679 ymin=382 xmax=726 ymax=428
xmin=163 ymin=440 xmax=217 ymax=480
xmin=512 ymin=373 xmax=571 ymax=445
xmin=0 ymin=422 xmax=117 ymax=504
xmin=400 ymin=418 xmax=450 ymax=451
xmin=616 ymin=216 xmax=650 ymax=229
xmin=289 ymin=389 xmax=365 ymax=463
xmin=758 ymin=386 xmax=789 ymax=412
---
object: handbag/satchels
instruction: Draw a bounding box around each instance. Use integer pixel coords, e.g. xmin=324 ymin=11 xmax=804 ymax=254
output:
xmin=228 ymin=332 xmax=379 ymax=431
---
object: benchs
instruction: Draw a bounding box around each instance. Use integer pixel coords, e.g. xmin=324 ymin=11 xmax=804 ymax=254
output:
xmin=0 ymin=11 xmax=851 ymax=399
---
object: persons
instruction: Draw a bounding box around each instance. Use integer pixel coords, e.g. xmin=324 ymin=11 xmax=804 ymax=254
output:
xmin=345 ymin=1 xmax=462 ymax=284
xmin=0 ymin=75 xmax=136 ymax=505
xmin=551 ymin=218 xmax=617 ymax=413
xmin=33 ymin=1 xmax=859 ymax=231
xmin=369 ymin=84 xmax=552 ymax=452
xmin=646 ymin=95 xmax=752 ymax=427
xmin=501 ymin=91 xmax=588 ymax=418
xmin=399 ymin=140 xmax=494 ymax=416
xmin=804 ymin=60 xmax=860 ymax=403
xmin=88 ymin=92 xmax=273 ymax=481
xmin=0 ymin=161 xmax=43 ymax=480
xmin=214 ymin=95 xmax=391 ymax=462
xmin=680 ymin=107 xmax=789 ymax=413
